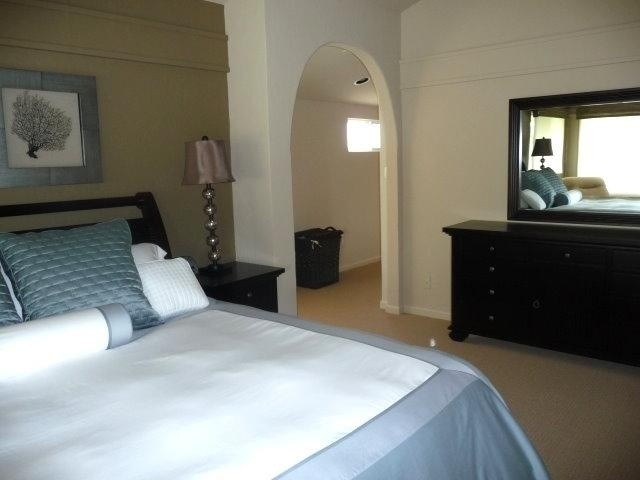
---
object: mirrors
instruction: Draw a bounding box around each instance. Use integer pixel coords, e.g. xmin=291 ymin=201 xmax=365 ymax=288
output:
xmin=505 ymin=85 xmax=640 ymax=228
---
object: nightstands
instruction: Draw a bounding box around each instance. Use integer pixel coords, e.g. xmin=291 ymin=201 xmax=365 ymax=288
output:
xmin=194 ymin=260 xmax=286 ymax=314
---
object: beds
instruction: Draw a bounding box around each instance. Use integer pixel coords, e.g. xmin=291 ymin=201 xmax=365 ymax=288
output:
xmin=0 ymin=189 xmax=501 ymax=480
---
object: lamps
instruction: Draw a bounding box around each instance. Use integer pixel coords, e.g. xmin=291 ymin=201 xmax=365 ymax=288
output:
xmin=531 ymin=136 xmax=553 ymax=169
xmin=178 ymin=133 xmax=235 ymax=277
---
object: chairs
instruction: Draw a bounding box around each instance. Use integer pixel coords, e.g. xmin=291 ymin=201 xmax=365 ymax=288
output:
xmin=562 ymin=175 xmax=610 ymax=201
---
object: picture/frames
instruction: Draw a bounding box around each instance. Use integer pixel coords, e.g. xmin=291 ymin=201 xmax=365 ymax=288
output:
xmin=0 ymin=66 xmax=105 ymax=187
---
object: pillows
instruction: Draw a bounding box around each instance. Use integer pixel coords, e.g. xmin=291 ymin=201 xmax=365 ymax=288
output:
xmin=0 ymin=216 xmax=209 ymax=387
xmin=516 ymin=166 xmax=584 ymax=211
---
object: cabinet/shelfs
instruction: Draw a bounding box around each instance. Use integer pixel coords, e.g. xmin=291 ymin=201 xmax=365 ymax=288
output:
xmin=437 ymin=219 xmax=640 ymax=366
